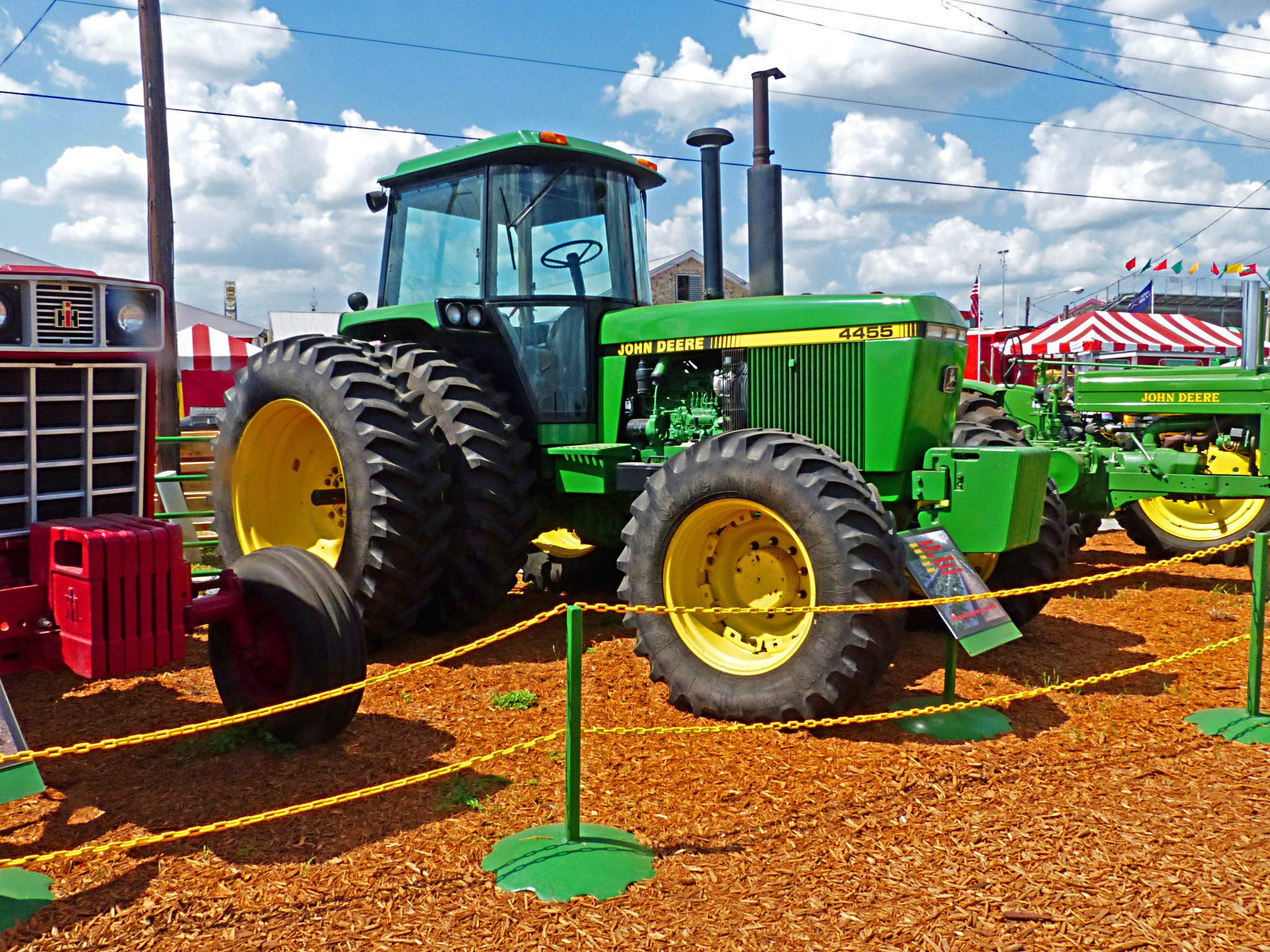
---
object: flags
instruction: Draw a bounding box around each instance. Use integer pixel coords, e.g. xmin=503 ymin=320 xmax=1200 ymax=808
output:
xmin=969 ymin=275 xmax=981 ymax=319
xmin=1125 ymin=257 xmax=1136 ymax=271
xmin=1210 ymin=262 xmax=1220 ymax=275
xmin=1225 ymin=263 xmax=1243 ymax=274
xmin=1188 ymin=259 xmax=1198 ymax=275
xmin=1217 ymin=262 xmax=1227 ymax=279
xmin=1139 ymin=258 xmax=1152 ymax=273
xmin=1238 ymin=263 xmax=1256 ymax=277
xmin=1171 ymin=260 xmax=1183 ymax=274
xmin=1126 ymin=280 xmax=1152 ymax=314
xmin=1152 ymin=258 xmax=1167 ymax=270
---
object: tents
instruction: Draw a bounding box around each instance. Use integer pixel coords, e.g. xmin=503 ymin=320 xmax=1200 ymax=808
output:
xmin=176 ymin=321 xmax=265 ymax=417
xmin=990 ymin=310 xmax=1270 ymax=395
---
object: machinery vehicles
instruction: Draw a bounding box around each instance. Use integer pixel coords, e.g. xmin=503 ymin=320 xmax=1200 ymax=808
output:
xmin=195 ymin=67 xmax=1066 ymax=721
xmin=1 ymin=261 xmax=367 ymax=748
xmin=954 ymin=278 xmax=1268 ymax=637
